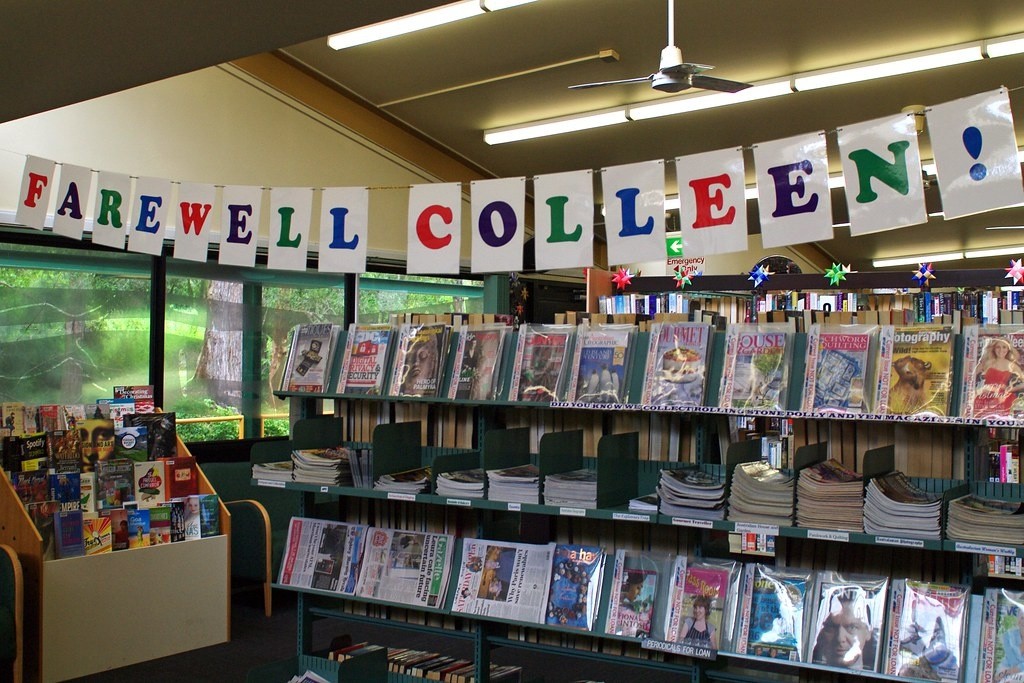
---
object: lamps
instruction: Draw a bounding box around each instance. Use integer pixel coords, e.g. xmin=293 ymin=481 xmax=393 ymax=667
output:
xmin=482 ymin=30 xmax=1024 ymax=147
xmin=327 ymin=0 xmax=535 ymax=51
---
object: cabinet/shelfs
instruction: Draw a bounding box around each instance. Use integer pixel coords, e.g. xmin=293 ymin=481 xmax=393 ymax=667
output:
xmin=0 ymin=402 xmax=230 ymax=683
xmin=250 ymin=267 xmax=1024 ymax=683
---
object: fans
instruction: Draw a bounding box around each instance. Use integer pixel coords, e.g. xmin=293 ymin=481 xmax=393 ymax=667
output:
xmin=568 ymin=0 xmax=754 ymax=94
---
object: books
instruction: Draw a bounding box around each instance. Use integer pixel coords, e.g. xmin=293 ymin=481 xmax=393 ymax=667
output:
xmin=0 ymin=291 xmax=1024 ymax=681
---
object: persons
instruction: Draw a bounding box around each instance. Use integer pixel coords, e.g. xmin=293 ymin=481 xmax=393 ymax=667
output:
xmin=618 ymin=571 xmax=648 ymax=639
xmin=819 ymin=591 xmax=872 ymax=671
xmin=974 ymin=336 xmax=1024 ymax=420
xmin=680 ymin=594 xmax=717 ymax=651
